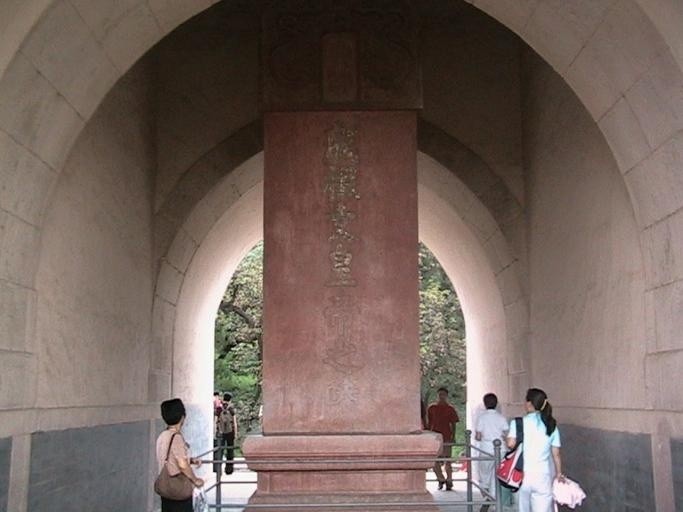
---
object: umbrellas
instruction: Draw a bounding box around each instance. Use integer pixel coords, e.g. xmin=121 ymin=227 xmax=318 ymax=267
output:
xmin=551 ymin=475 xmax=586 ymax=510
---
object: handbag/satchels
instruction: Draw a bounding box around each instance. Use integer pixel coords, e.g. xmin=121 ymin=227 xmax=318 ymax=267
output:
xmin=154 ymin=465 xmax=193 ymax=500
xmin=497 ymin=445 xmax=523 ymax=490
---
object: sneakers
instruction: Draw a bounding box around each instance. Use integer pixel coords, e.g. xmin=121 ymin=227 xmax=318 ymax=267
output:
xmin=438 ymin=481 xmax=452 ymax=490
xmin=478 ymin=496 xmax=491 ymax=512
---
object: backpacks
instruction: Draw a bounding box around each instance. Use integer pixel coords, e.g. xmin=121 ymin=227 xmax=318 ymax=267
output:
xmin=218 ymin=405 xmax=233 ymax=434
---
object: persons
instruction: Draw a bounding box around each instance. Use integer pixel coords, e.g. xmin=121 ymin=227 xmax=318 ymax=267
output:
xmin=504 ymin=388 xmax=565 ymax=512
xmin=213 ymin=392 xmax=238 ymax=475
xmin=154 ymin=397 xmax=203 ymax=512
xmin=420 ymin=399 xmax=427 ymax=430
xmin=473 ymin=393 xmax=509 ymax=512
xmin=213 ymin=391 xmax=222 ymax=473
xmin=425 ymin=387 xmax=458 ymax=491
